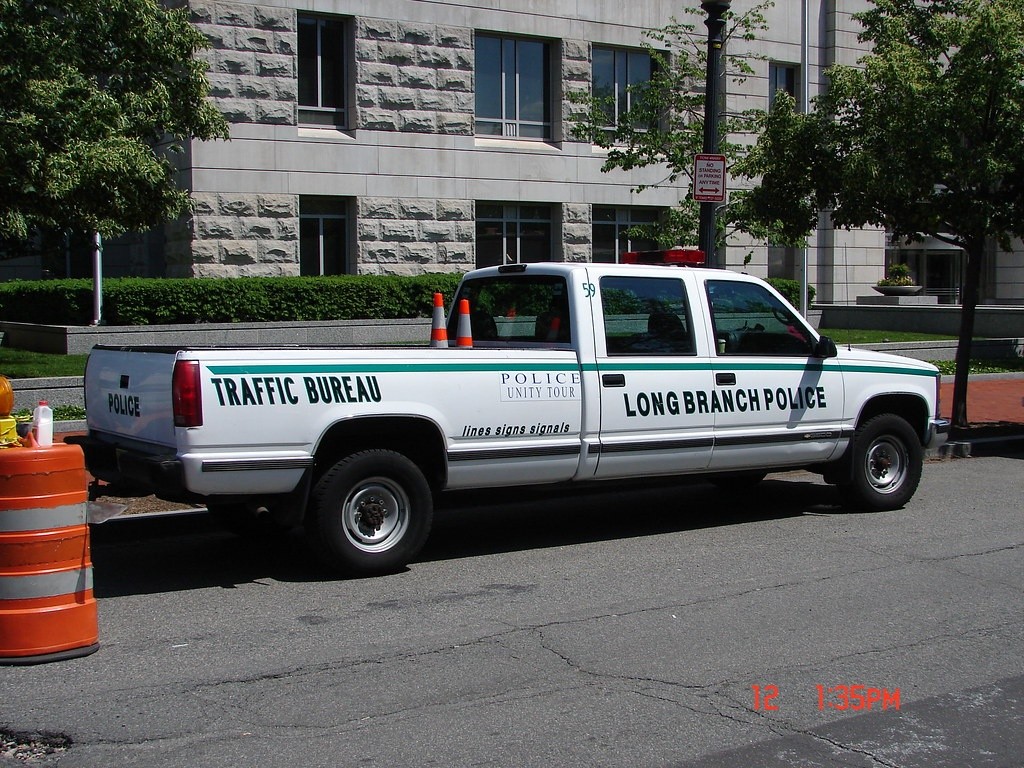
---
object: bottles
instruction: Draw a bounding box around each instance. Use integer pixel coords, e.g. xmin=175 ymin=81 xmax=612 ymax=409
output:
xmin=31 ymin=401 xmax=54 ymax=445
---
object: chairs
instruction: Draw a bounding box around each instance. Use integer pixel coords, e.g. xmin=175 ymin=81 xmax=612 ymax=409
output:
xmin=647 ymin=309 xmax=686 ymax=337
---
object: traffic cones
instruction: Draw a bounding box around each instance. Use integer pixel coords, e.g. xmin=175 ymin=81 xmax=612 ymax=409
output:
xmin=454 ymin=298 xmax=473 ymax=348
xmin=427 ymin=292 xmax=449 ymax=349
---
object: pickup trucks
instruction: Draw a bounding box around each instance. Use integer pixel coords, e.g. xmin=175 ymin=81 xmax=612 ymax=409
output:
xmin=63 ymin=257 xmax=952 ymax=577
